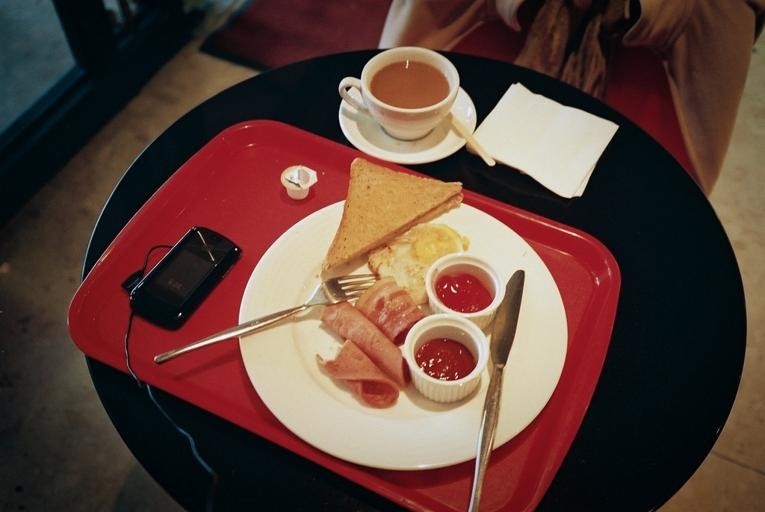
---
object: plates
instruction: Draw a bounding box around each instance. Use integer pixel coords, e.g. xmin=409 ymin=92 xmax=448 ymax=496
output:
xmin=236 ymin=197 xmax=572 ymax=477
xmin=338 ymin=79 xmax=480 ymax=166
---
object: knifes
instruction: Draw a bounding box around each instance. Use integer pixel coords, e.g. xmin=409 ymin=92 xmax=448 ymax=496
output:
xmin=463 ymin=267 xmax=530 ymax=512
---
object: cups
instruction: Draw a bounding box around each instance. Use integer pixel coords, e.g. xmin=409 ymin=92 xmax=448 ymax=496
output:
xmin=399 ymin=312 xmax=489 ymax=405
xmin=339 ymin=45 xmax=461 ymax=142
xmin=423 ymin=250 xmax=505 ymax=332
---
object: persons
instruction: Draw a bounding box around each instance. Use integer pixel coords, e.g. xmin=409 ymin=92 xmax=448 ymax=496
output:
xmin=376 ymin=0 xmax=757 ymax=202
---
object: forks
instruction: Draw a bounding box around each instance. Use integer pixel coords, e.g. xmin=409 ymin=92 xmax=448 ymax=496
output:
xmin=152 ymin=268 xmax=380 ymax=368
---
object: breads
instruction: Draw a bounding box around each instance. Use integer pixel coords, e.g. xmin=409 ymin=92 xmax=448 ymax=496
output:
xmin=321 ymin=157 xmax=464 ymax=271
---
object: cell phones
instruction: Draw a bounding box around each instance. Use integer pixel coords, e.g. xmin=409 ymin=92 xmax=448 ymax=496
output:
xmin=130 ymin=226 xmax=240 ymax=330
